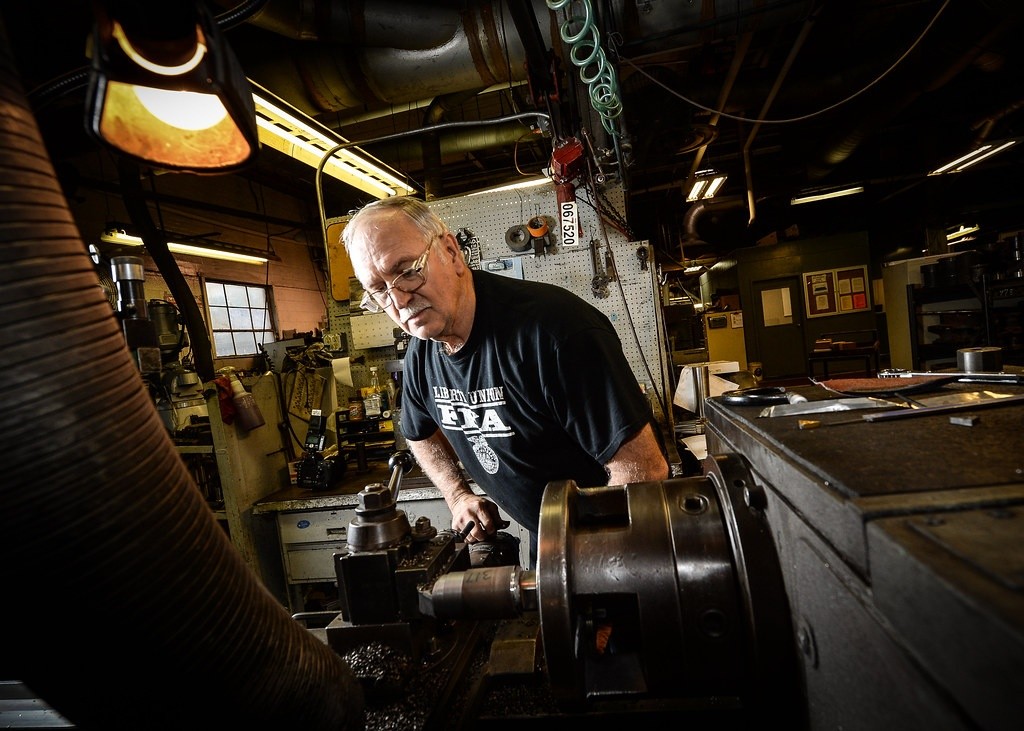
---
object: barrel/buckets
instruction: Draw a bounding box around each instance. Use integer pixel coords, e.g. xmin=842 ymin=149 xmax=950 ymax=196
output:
xmin=748 ymin=362 xmax=762 ymax=381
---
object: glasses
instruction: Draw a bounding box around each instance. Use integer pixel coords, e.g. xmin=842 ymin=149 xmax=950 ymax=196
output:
xmin=359 ymin=233 xmax=438 ymax=313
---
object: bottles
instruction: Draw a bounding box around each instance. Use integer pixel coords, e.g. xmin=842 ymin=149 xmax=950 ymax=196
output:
xmin=367 ymin=367 xmax=382 ymax=407
xmin=386 ymin=379 xmax=396 ymax=411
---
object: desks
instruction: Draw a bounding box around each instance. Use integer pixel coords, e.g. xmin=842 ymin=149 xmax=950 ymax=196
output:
xmin=807 ymin=347 xmax=874 ymax=386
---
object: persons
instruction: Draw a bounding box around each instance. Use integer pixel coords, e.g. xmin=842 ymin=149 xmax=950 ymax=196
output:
xmin=339 ymin=197 xmax=670 ymax=545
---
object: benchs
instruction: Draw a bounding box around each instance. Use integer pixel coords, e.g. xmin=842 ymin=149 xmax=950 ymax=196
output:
xmin=821 ymin=329 xmax=880 ymax=369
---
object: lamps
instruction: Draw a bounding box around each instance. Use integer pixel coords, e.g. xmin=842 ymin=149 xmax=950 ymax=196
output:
xmin=77 ymin=0 xmax=261 ymax=385
xmin=685 ymin=173 xmax=728 ymax=202
xmin=243 ymin=74 xmax=420 ymax=201
xmin=99 ymin=222 xmax=282 ymax=266
xmin=925 ymin=138 xmax=1022 ymax=177
xmin=790 ymin=181 xmax=863 ymax=205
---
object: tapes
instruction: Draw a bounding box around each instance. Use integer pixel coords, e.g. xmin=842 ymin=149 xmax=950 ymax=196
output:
xmin=505 ymin=225 xmax=532 ymax=252
xmin=957 ymin=347 xmax=1004 ymax=373
xmin=527 ymin=216 xmax=547 ymax=237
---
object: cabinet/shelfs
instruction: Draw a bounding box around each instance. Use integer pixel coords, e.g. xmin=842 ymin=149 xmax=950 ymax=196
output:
xmin=702 ymin=365 xmax=1024 ymax=731
xmin=256 ymin=462 xmax=534 ymax=627
xmin=175 ymin=375 xmax=285 ymax=593
xmin=907 ymin=271 xmax=1024 ymax=373
xmin=656 ymin=262 xmax=708 ymax=368
xmin=880 ymin=251 xmax=965 ymax=371
